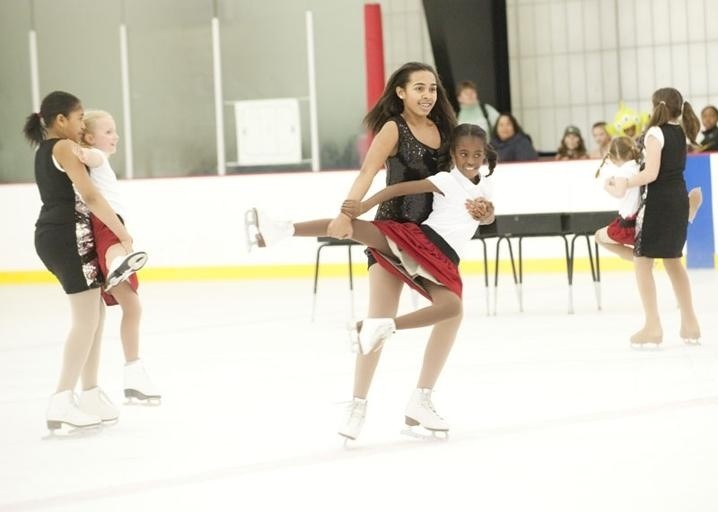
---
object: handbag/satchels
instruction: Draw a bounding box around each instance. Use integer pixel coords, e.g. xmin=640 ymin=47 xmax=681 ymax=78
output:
xmin=608 ymin=214 xmax=636 ymax=245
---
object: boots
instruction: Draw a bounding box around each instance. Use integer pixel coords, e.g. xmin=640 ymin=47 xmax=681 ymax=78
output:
xmin=358 ymin=318 xmax=396 ymax=354
xmin=125 ymin=360 xmax=161 ymax=399
xmin=255 ymin=209 xmax=295 ymax=247
xmin=46 ymin=387 xmax=117 ymax=425
xmin=406 ymin=388 xmax=447 ymax=431
xmin=339 ymin=398 xmax=368 ymax=439
xmin=105 ymin=251 xmax=147 ymax=286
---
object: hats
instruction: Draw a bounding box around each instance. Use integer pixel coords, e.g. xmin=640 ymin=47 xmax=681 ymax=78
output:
xmin=565 ymin=127 xmax=579 ymax=136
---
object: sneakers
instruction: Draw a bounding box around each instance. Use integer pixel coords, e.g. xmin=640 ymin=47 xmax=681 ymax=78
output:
xmin=632 ymin=329 xmax=700 ymax=342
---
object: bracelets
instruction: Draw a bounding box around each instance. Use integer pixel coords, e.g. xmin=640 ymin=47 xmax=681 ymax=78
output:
xmin=626 ymin=177 xmax=630 ymax=188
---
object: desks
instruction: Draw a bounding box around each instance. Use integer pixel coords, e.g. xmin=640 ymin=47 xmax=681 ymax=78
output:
xmin=313 ymin=212 xmax=619 ymax=296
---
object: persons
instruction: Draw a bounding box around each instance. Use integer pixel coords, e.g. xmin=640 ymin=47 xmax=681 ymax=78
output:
xmin=607 ymin=88 xmax=701 ymax=344
xmin=698 ymin=106 xmax=718 ymax=151
xmin=593 ymin=122 xmax=612 ymax=160
xmin=328 ymin=62 xmax=495 ymax=438
xmin=252 ymin=124 xmax=498 ymax=355
xmin=595 ymin=136 xmax=643 ymax=262
xmin=456 ymin=81 xmax=500 ymax=162
xmin=553 ymin=126 xmax=592 ymax=161
xmin=65 ymin=109 xmax=162 ymax=401
xmin=489 ymin=114 xmax=541 ymax=162
xmin=25 ymin=92 xmax=133 ymax=430
